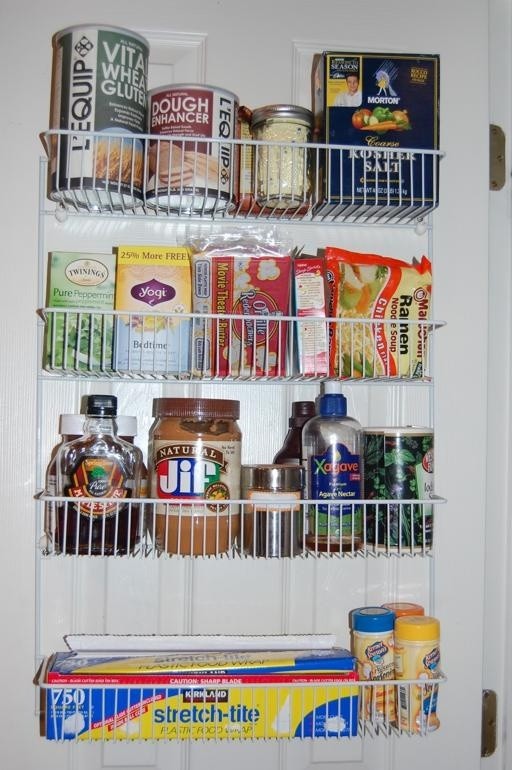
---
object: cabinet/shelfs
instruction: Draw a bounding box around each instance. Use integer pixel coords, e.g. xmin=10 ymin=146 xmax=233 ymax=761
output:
xmin=35 ymin=128 xmax=447 ymax=745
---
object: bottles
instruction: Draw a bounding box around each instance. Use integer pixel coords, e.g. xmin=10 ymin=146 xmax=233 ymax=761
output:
xmin=43 ymin=392 xmax=149 ymax=556
xmin=249 ymin=104 xmax=313 ymax=210
xmin=303 ymin=392 xmax=363 ymax=553
xmin=238 ymin=465 xmax=302 ymax=557
xmin=272 ymin=401 xmax=328 ymax=466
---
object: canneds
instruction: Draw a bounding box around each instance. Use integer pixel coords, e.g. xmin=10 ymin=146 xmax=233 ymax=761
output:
xmin=148 ymin=83 xmax=239 ymax=214
xmin=46 ymin=22 xmax=149 ymax=209
xmin=149 ymin=395 xmax=244 ymax=553
xmin=360 ymin=425 xmax=435 ymax=553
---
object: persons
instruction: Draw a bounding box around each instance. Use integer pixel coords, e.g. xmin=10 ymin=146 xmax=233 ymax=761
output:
xmin=334 ymin=72 xmax=362 ymax=107
